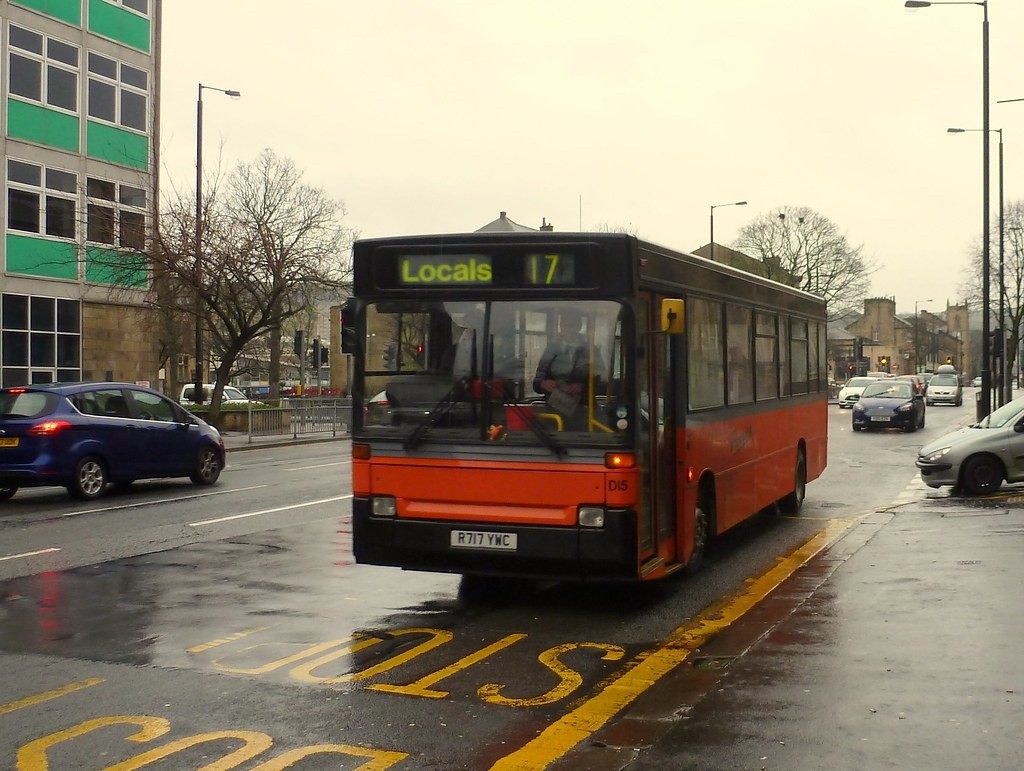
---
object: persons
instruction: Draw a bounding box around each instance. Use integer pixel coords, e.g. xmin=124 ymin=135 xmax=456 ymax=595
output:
xmin=724 ymin=346 xmax=750 ymax=401
xmin=532 ymin=307 xmax=606 ymax=432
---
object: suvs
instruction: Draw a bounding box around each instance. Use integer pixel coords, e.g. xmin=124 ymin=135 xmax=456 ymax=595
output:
xmin=179 ymin=384 xmax=264 ymax=407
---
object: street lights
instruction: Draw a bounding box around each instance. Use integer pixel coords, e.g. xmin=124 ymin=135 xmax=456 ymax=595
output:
xmin=947 ymin=127 xmax=1004 ymax=409
xmin=195 ymin=82 xmax=241 ymax=405
xmin=711 ymin=201 xmax=747 ymax=261
xmin=915 ymin=299 xmax=933 ymax=374
xmin=905 ymin=0 xmax=991 ymax=424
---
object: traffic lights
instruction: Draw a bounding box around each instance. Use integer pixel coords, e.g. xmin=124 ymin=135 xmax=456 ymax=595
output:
xmin=946 ymin=355 xmax=952 ymax=365
xmin=881 ymin=356 xmax=890 ymax=373
xmin=988 ymin=326 xmax=1001 ymax=358
xmin=848 ymin=363 xmax=855 ymax=373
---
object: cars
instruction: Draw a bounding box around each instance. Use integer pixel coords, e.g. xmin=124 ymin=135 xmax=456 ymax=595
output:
xmin=972 ymin=376 xmax=983 ymax=388
xmin=925 ymin=365 xmax=965 ymax=407
xmin=0 ymin=381 xmax=226 ymax=503
xmin=866 ymin=371 xmax=934 ymax=395
xmin=839 ymin=376 xmax=882 ymax=408
xmin=232 ymin=380 xmax=348 ymax=402
xmin=915 ymin=395 xmax=1024 ymax=497
xmin=852 ymin=379 xmax=926 ymax=432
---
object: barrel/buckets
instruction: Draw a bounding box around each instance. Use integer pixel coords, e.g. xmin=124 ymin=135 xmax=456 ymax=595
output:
xmin=504 ymin=403 xmax=534 ymax=430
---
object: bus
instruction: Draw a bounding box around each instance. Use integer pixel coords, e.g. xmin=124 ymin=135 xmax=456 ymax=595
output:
xmin=340 ymin=217 xmax=829 ymax=585
